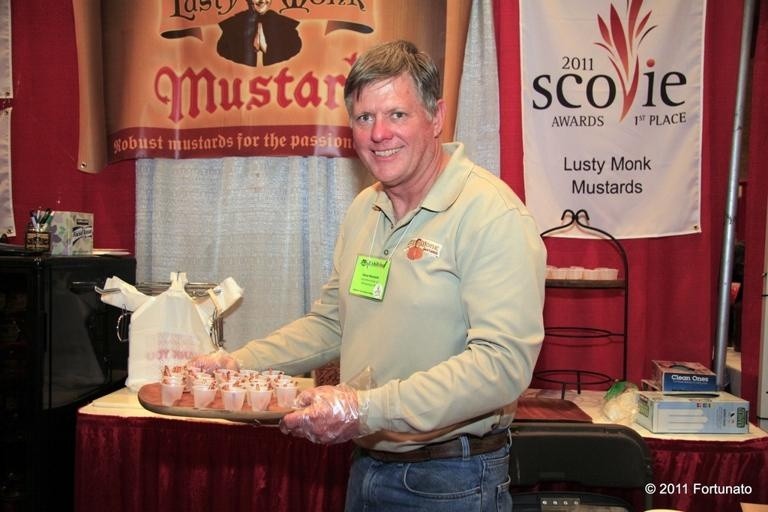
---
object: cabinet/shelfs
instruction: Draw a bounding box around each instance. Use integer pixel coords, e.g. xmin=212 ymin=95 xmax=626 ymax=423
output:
xmin=537 ymin=209 xmax=630 ymax=398
xmin=0 ymin=246 xmax=136 ymax=511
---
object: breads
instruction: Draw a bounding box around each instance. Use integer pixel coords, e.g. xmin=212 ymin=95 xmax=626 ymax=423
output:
xmin=601 ymin=389 xmax=640 ymax=428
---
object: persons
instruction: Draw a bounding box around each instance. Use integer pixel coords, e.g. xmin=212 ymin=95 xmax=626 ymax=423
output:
xmin=187 ymin=39 xmax=549 ymax=512
xmin=216 ymin=0 xmax=302 ymax=66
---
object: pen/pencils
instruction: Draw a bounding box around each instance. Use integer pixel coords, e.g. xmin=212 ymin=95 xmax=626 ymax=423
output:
xmin=29 ymin=206 xmax=55 ymax=232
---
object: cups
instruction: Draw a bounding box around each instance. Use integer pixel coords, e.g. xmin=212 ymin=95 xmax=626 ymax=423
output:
xmin=159 ymin=369 xmax=299 ymax=412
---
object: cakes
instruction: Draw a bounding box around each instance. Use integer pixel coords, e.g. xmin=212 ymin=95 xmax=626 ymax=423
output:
xmin=546 ymin=264 xmax=620 ymax=281
xmin=160 ymin=363 xmax=301 ymax=412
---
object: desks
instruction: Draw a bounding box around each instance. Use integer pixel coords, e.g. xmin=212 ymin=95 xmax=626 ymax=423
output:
xmin=77 ymin=361 xmax=767 ymax=512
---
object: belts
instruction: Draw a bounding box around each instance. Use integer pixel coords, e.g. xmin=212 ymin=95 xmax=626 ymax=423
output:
xmin=356 ymin=429 xmax=510 ymax=466
